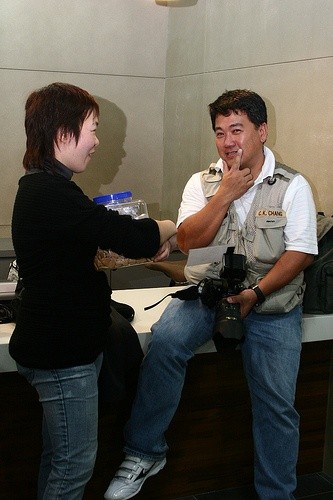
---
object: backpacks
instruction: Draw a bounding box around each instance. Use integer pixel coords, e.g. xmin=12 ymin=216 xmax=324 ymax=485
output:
xmin=300 ymin=215 xmax=333 ymax=316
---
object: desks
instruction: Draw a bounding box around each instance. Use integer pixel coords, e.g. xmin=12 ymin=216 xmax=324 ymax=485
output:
xmin=0 ymin=281 xmax=331 ymax=500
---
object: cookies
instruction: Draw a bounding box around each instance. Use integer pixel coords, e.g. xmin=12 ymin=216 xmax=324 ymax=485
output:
xmin=95 ymin=248 xmax=147 ymax=269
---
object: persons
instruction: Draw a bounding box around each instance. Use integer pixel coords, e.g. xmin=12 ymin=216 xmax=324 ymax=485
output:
xmin=8 ymin=81 xmax=178 ymax=500
xmin=104 ymin=90 xmax=319 ymax=500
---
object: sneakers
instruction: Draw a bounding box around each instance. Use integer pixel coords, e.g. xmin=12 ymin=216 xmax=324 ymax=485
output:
xmin=104 ymin=456 xmax=166 ymax=500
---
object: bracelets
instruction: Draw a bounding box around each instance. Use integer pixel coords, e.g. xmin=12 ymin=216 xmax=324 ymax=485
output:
xmin=248 ymin=284 xmax=268 ymax=307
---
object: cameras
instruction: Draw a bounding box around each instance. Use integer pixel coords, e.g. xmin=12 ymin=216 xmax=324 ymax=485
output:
xmin=200 ymin=251 xmax=248 ymax=357
